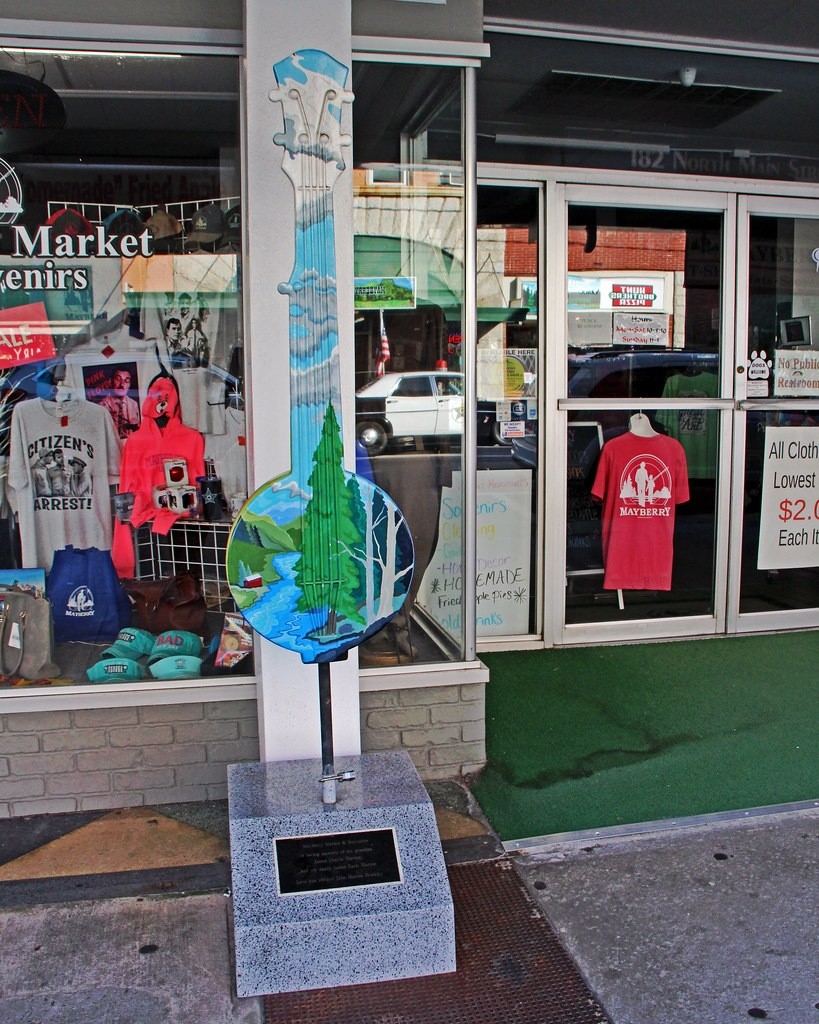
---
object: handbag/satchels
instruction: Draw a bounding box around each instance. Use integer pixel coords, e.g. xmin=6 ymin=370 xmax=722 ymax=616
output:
xmin=1 ymin=544 xmax=207 ymax=682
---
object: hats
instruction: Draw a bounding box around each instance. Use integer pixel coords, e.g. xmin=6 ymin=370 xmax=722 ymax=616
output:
xmin=45 ymin=203 xmax=242 ymax=255
xmin=86 ymin=627 xmax=205 ymax=685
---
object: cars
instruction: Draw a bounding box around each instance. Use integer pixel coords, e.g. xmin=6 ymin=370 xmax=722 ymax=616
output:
xmin=355 ymin=371 xmax=522 ymax=457
xmin=511 ymin=346 xmax=721 ymax=488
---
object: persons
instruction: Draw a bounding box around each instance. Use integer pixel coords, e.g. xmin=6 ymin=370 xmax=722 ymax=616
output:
xmin=161 ymin=292 xmax=214 ymax=369
xmin=98 ymin=366 xmax=140 ymax=439
xmin=30 ymin=447 xmax=53 ymax=498
xmin=47 ymin=449 xmax=73 ymax=497
xmin=68 ymin=456 xmax=92 ymax=497
